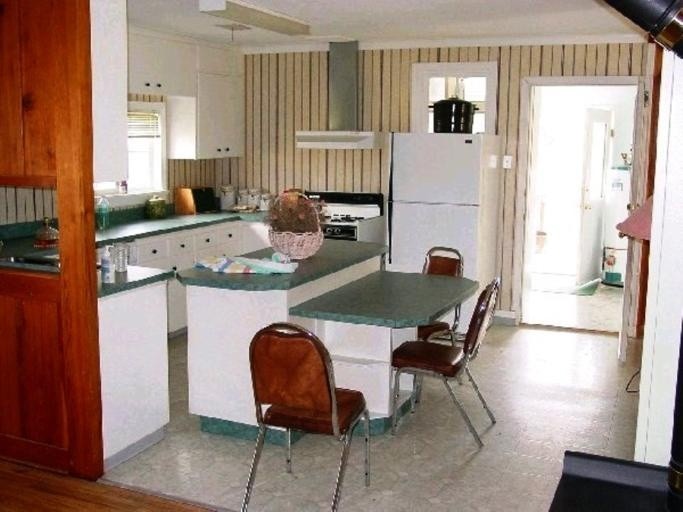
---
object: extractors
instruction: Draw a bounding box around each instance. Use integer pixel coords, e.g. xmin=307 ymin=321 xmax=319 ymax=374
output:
xmin=297 ymin=41 xmax=385 ymax=152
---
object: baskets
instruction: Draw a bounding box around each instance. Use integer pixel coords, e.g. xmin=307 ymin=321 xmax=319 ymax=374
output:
xmin=268 ymin=192 xmax=324 ymax=260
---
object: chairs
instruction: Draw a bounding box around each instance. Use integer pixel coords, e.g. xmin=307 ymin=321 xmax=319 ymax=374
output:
xmin=391 ymin=277 xmax=501 ymax=447
xmin=240 ymin=321 xmax=371 ymax=512
xmin=418 ymin=247 xmax=464 ymax=404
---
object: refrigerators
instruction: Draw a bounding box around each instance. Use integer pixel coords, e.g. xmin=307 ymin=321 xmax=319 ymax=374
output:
xmin=381 ymin=129 xmax=504 ymax=335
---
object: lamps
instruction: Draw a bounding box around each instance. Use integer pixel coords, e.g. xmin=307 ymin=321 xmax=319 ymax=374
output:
xmin=616 ymin=195 xmax=653 ymax=394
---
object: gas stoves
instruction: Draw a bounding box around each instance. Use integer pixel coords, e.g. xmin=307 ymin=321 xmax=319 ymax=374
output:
xmin=315 ymin=215 xmax=361 ymax=242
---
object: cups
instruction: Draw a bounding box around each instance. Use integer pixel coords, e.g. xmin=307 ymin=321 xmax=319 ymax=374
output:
xmin=114 ymin=246 xmax=127 ymax=272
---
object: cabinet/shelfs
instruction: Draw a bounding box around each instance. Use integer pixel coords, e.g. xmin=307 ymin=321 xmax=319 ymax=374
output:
xmin=108 ymin=221 xmax=241 ymax=334
xmin=90 ymin=0 xmax=129 ymax=184
xmin=165 ymin=25 xmax=245 ymax=160
xmin=127 ymin=24 xmax=198 ymax=101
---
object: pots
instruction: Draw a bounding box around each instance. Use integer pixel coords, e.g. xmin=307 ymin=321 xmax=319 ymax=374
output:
xmin=425 ymin=95 xmax=479 ymax=134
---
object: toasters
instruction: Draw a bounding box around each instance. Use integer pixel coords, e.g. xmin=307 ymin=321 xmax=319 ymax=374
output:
xmin=174 ymin=185 xmax=216 ymax=214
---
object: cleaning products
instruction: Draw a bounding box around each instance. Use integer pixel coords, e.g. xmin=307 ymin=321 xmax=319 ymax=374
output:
xmin=96 ymin=193 xmax=111 ymax=229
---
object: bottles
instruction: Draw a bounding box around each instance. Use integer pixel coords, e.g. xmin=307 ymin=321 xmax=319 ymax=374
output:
xmin=119 ymin=180 xmax=127 ymax=194
xmin=95 ymin=193 xmax=109 ymax=228
xmin=219 ymin=184 xmax=271 ymax=212
